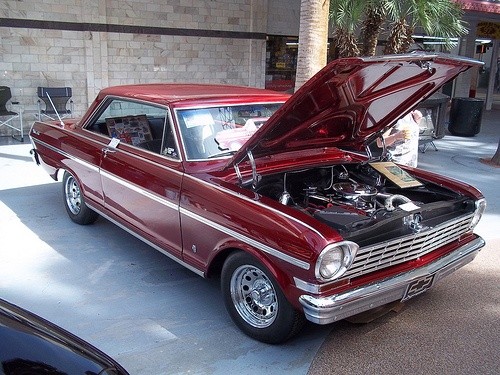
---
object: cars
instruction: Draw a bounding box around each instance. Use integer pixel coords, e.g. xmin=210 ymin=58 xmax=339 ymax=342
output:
xmin=27 ymin=50 xmax=487 ymax=344
xmin=0 ymin=299 xmax=131 ymax=375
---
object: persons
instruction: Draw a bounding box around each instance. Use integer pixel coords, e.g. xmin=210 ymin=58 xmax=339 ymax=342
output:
xmin=369 ymin=110 xmax=423 ymax=169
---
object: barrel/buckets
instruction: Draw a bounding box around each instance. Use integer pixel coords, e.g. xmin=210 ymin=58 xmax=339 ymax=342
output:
xmin=448 ymin=97 xmax=485 ymax=137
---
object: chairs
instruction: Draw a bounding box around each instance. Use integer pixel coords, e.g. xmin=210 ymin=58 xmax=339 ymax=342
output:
xmin=136 ymin=138 xmax=167 ymax=154
xmin=0 ymin=86 xmax=25 ymax=143
xmin=32 ymin=87 xmax=74 ymax=121
